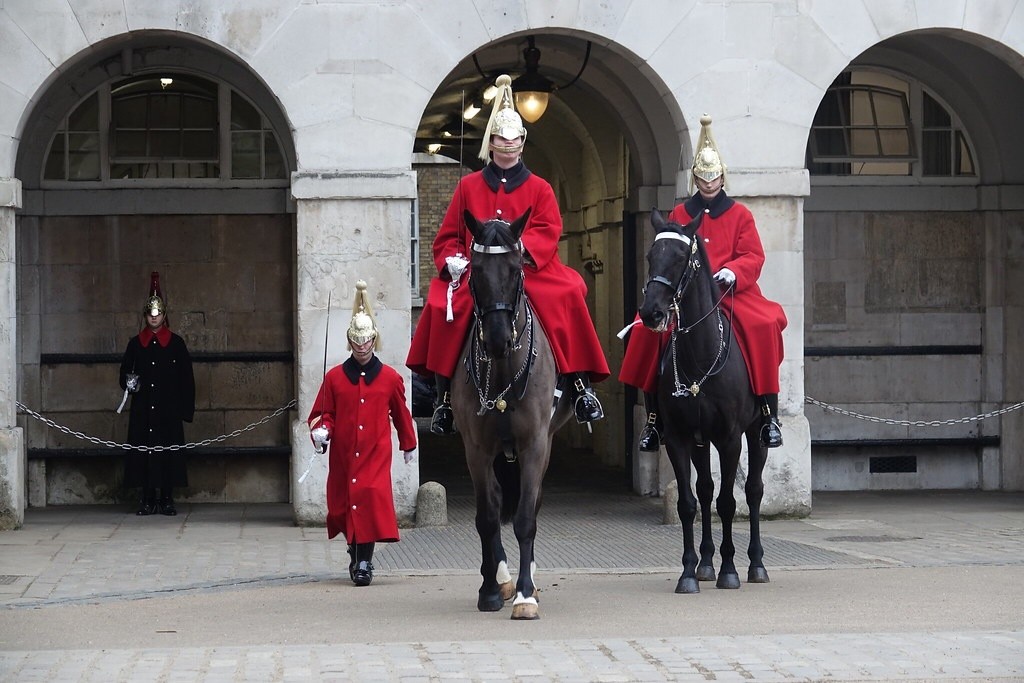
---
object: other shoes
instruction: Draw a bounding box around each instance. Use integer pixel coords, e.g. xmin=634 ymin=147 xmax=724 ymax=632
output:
xmin=355 ymin=569 xmax=372 ymax=584
xmin=350 ymin=562 xmax=357 ymax=582
xmin=137 ymin=501 xmax=157 ymax=515
xmin=159 ymin=499 xmax=176 ymax=516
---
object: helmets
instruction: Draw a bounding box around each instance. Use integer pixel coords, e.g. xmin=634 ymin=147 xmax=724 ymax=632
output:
xmin=143 ymin=271 xmax=169 ymax=326
xmin=478 ymin=75 xmax=526 ymax=164
xmin=687 ymin=113 xmax=727 ymax=196
xmin=346 ymin=279 xmax=379 ymax=352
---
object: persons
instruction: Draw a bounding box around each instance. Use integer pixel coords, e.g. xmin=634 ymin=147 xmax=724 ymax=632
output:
xmin=408 ymin=111 xmax=611 ymax=434
xmin=309 ymin=327 xmax=417 ymax=583
xmin=120 ymin=298 xmax=196 ymax=516
xmin=618 ymin=155 xmax=788 ymax=453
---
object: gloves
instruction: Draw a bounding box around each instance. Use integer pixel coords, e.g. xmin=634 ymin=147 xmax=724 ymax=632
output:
xmin=713 ymin=268 xmax=736 ymax=285
xmin=403 ymin=449 xmax=416 ymax=464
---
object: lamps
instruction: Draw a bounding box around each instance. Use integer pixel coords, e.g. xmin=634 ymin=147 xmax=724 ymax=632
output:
xmin=483 ymin=84 xmax=498 ymax=100
xmin=160 ymin=77 xmax=173 ymax=84
xmin=428 ymin=143 xmax=442 ymax=155
xmin=463 ymin=100 xmax=482 ymax=119
xmin=512 ymin=33 xmax=558 ymax=122
xmin=445 ymin=132 xmax=451 ymax=137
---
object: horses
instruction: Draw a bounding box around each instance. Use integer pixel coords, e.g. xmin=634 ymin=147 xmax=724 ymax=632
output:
xmin=640 ymin=212 xmax=778 ymax=593
xmin=449 ymin=207 xmax=574 ymax=619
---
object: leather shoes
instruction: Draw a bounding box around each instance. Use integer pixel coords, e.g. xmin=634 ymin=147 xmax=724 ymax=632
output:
xmin=641 ymin=424 xmax=663 ymax=449
xmin=432 ymin=405 xmax=453 ymax=433
xmin=574 ymin=388 xmax=601 ymax=421
xmin=763 ymin=414 xmax=781 ymax=445
